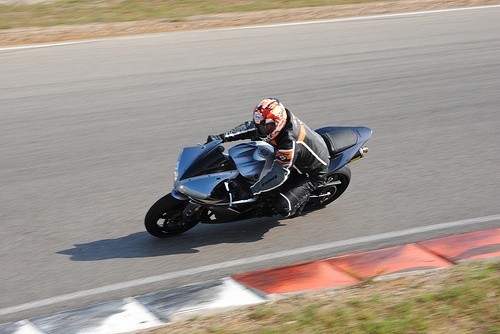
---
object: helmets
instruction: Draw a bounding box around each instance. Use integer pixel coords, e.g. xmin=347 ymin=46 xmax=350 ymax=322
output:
xmin=253 ymin=97 xmax=287 ymax=141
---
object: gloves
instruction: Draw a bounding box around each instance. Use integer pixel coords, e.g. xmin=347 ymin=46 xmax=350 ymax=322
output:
xmin=231 ymin=188 xmax=251 ymax=202
xmin=204 ymin=135 xmax=221 ymax=144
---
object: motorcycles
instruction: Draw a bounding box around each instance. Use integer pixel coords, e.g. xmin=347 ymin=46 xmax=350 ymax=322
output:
xmin=145 ymin=125 xmax=372 ymax=237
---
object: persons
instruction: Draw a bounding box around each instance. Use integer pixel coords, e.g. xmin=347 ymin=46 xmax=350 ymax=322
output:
xmin=205 ymin=98 xmax=330 ymax=218
